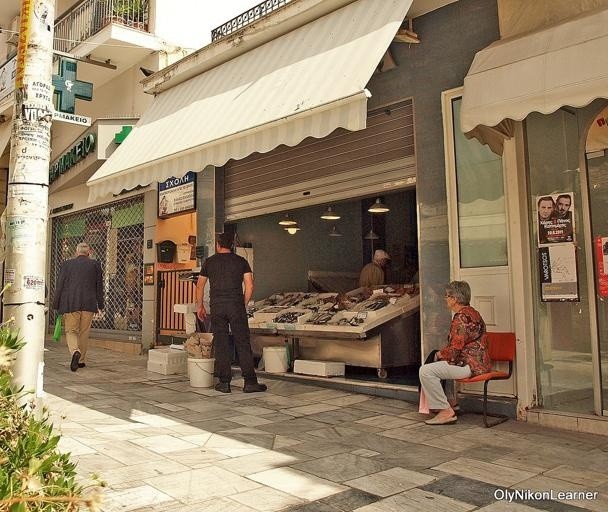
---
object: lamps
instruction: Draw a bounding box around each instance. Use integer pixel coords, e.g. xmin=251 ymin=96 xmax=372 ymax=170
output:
xmin=278 ymin=198 xmax=390 ymax=234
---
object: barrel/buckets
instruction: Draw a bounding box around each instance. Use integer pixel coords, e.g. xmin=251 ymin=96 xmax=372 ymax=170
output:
xmin=263 ymin=346 xmax=287 ymax=373
xmin=187 ymin=358 xmax=215 ymax=388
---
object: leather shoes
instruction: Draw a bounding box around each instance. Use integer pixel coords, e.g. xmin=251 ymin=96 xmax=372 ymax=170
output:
xmin=423 ymin=414 xmax=457 ymax=425
xmin=77 ymin=363 xmax=85 ymax=368
xmin=70 ymin=351 xmax=80 ymax=371
xmin=431 ymin=403 xmax=460 ymax=413
xmin=242 ymin=384 xmax=267 ymax=393
xmin=214 ymin=383 xmax=230 ymax=393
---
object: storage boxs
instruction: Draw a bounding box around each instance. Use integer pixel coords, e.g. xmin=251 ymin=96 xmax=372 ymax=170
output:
xmin=294 ymin=359 xmax=346 ymax=377
xmin=147 ymin=348 xmax=187 ymax=376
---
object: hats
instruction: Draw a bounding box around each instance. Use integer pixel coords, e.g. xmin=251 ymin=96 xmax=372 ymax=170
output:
xmin=373 ymin=249 xmax=392 ymax=261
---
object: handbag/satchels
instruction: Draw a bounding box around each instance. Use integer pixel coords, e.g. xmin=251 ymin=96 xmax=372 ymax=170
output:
xmin=51 ymin=313 xmax=61 ymax=342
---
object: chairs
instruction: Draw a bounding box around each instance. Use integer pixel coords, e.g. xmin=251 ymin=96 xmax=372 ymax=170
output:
xmin=455 ymin=332 xmax=516 ymax=428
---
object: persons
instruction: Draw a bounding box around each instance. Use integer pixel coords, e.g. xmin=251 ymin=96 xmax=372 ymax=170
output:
xmin=52 ymin=242 xmax=104 ymax=371
xmin=419 ymin=281 xmax=491 ymax=425
xmin=195 ymin=233 xmax=266 ymax=393
xmin=359 ymin=249 xmax=391 ymax=288
xmin=538 ymin=194 xmax=572 ymax=244
